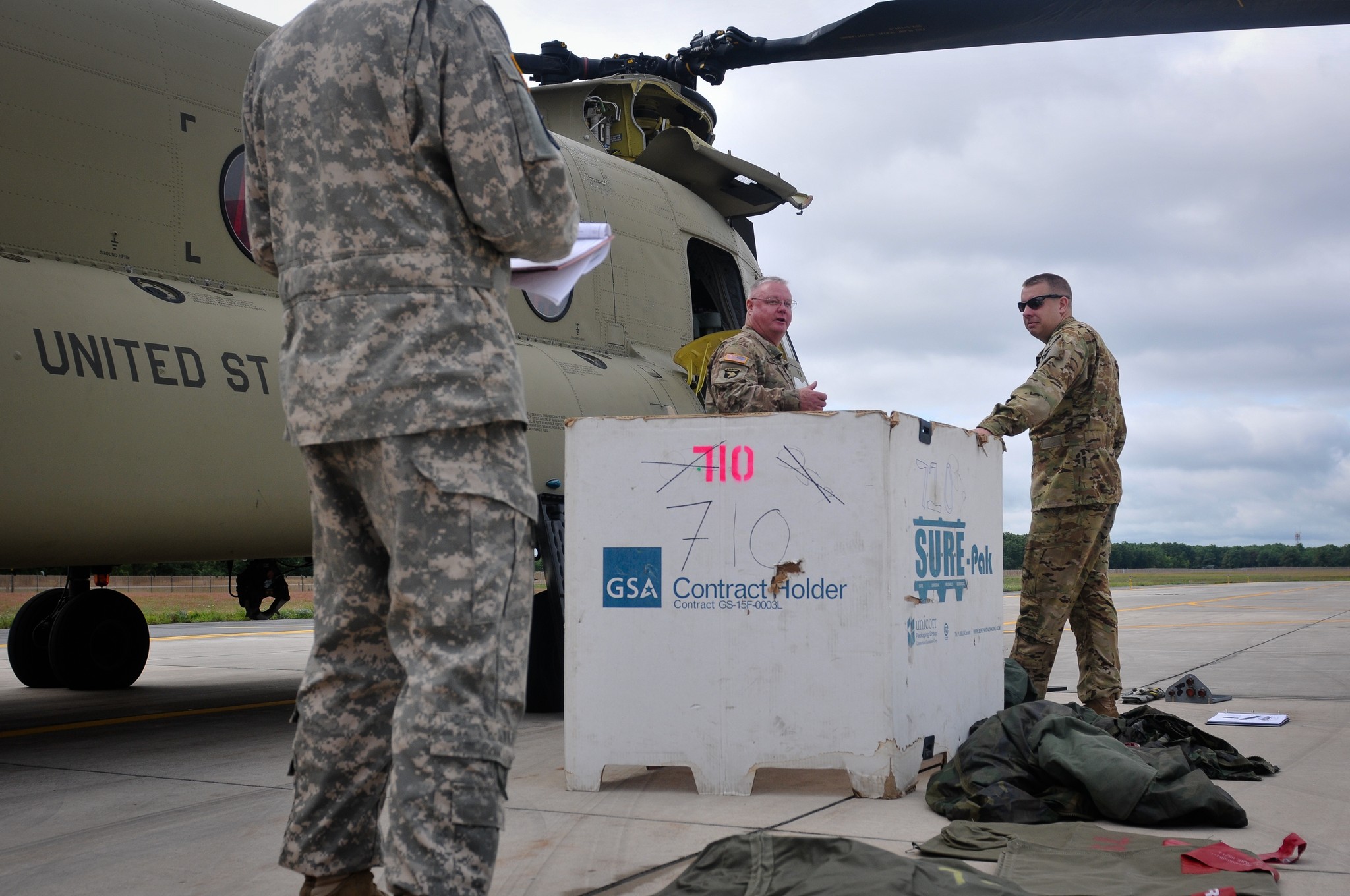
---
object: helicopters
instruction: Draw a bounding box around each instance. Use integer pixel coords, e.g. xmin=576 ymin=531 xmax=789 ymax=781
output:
xmin=0 ymin=1 xmax=1350 ymax=695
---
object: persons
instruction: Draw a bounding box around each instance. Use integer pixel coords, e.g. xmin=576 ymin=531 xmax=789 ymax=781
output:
xmin=243 ymin=0 xmax=580 ymax=896
xmin=705 ymin=277 xmax=827 ymax=413
xmin=976 ymin=273 xmax=1127 ymax=717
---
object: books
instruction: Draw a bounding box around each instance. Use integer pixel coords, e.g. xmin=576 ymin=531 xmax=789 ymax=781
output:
xmin=510 ymin=222 xmax=615 ymax=306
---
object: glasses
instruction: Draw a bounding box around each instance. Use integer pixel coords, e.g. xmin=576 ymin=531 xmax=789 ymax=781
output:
xmin=1017 ymin=294 xmax=1070 ymax=312
xmin=752 ymin=297 xmax=797 ymax=309
